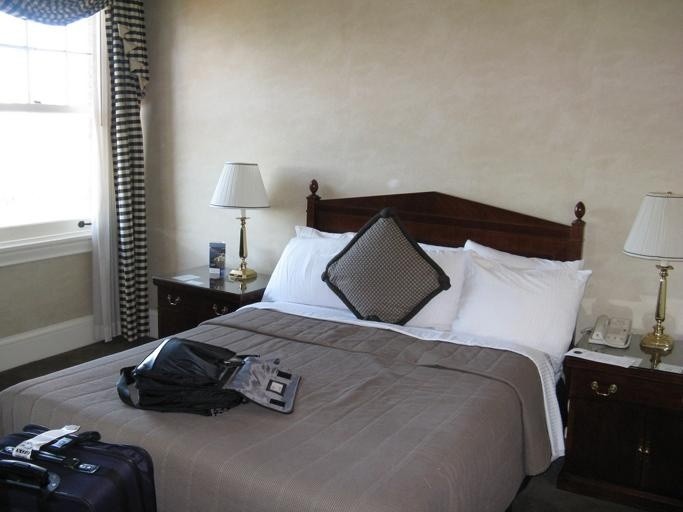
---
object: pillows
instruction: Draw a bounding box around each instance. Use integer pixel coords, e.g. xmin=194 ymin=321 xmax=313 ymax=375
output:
xmin=317 ymin=206 xmax=452 ymax=326
xmin=458 ymin=237 xmax=587 ymax=271
xmin=467 ymin=247 xmax=592 ymax=364
xmin=291 ymin=221 xmax=461 ymax=252
xmin=260 ymin=234 xmax=467 ymax=333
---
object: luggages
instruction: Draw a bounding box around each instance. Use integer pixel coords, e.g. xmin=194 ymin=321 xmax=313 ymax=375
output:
xmin=0 ymin=424 xmax=159 ymax=512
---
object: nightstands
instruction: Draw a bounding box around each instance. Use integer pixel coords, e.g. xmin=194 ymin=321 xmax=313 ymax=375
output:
xmin=552 ymin=326 xmax=683 ymax=508
xmin=150 ymin=262 xmax=272 ymax=340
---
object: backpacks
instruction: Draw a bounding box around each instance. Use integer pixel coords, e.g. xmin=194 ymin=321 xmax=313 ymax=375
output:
xmin=116 ymin=336 xmax=301 ymax=419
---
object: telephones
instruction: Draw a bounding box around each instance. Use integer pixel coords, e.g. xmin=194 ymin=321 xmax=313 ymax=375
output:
xmin=588 ymin=315 xmax=632 ymax=348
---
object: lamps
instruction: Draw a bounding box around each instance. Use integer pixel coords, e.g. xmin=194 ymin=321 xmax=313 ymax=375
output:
xmin=618 ymin=189 xmax=682 ymax=351
xmin=206 ymin=160 xmax=272 ymax=283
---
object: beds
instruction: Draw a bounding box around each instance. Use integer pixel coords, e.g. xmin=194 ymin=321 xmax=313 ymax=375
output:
xmin=1 ymin=179 xmax=592 ymax=511
